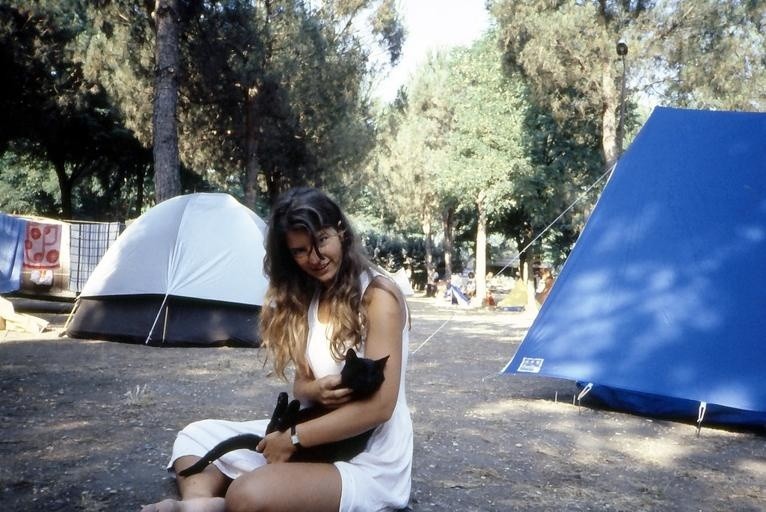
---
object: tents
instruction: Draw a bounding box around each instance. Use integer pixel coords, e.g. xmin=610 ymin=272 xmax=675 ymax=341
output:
xmin=56 ymin=190 xmax=295 ymax=349
xmin=481 ymin=104 xmax=766 ymax=434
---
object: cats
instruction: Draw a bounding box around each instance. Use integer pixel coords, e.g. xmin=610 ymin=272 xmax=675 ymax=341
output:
xmin=178 ymin=347 xmax=390 ymax=477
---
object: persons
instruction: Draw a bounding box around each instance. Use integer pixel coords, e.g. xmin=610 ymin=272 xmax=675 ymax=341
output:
xmin=536 ymin=268 xmax=554 ymax=304
xmin=428 ymin=267 xmax=439 ymax=297
xmin=486 ymin=271 xmax=494 ymax=286
xmin=466 ymin=272 xmax=477 ymax=297
xmin=140 ymin=184 xmax=415 ymax=512
xmin=448 ymin=272 xmax=464 ymax=290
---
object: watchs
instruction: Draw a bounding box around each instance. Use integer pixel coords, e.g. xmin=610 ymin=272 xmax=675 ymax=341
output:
xmin=290 ymin=424 xmax=305 ymax=453
